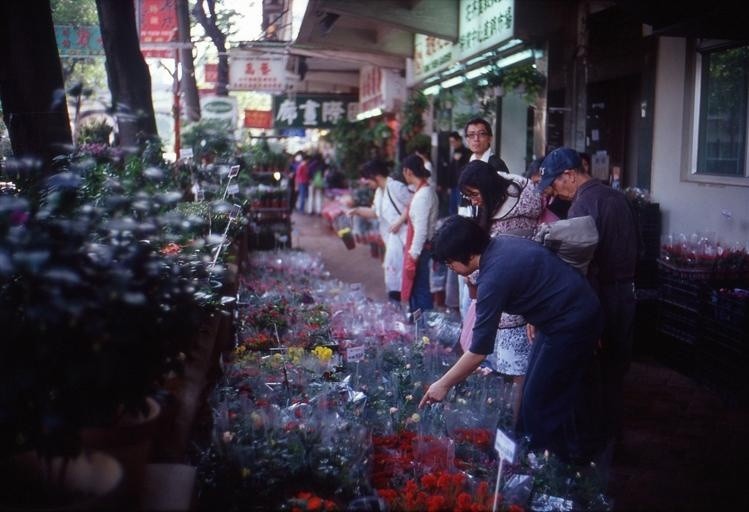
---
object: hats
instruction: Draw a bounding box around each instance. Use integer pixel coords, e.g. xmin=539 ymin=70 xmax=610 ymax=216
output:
xmin=533 ymin=148 xmax=580 ymax=192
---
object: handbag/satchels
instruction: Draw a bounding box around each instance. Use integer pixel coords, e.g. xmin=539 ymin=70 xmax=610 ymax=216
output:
xmin=532 ymin=215 xmax=599 ymax=273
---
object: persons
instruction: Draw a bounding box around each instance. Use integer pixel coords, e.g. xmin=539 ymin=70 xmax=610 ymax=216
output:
xmin=417 ymin=214 xmax=602 ymax=452
xmin=290 ymin=150 xmax=330 ymax=215
xmin=347 ymin=118 xmax=512 ymax=331
xmin=455 ymin=147 xmax=635 ymax=442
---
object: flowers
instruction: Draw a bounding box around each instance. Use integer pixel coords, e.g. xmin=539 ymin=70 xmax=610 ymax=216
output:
xmin=0 ymin=137 xmax=612 ymax=512
xmin=659 ymin=232 xmax=748 ymax=324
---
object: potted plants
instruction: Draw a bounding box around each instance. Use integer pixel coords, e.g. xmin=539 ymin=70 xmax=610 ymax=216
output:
xmin=373 ymin=60 xmax=547 ymax=139
